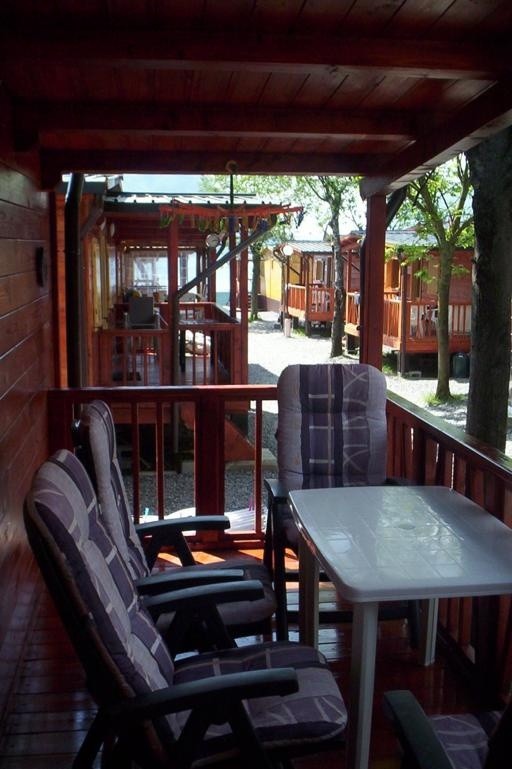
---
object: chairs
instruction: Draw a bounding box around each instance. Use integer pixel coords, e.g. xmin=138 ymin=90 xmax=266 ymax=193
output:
xmin=262 ymin=362 xmax=421 ymax=653
xmin=380 ymin=684 xmax=511 ymax=769
xmin=25 ymin=445 xmax=349 ymax=769
xmin=74 ymin=396 xmax=281 ymax=665
xmin=124 ymin=295 xmax=161 ymax=360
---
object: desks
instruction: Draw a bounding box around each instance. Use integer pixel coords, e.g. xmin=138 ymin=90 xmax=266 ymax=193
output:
xmin=286 ymin=486 xmax=510 ymax=769
